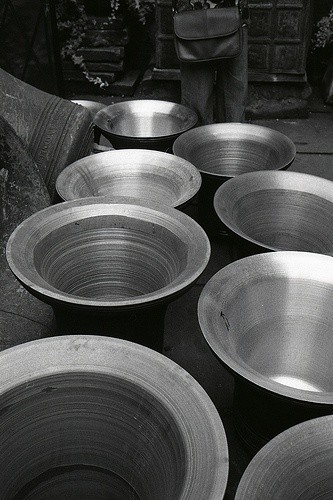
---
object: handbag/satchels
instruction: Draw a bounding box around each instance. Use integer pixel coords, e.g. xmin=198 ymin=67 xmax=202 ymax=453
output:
xmin=172 ymin=7 xmax=244 ymax=64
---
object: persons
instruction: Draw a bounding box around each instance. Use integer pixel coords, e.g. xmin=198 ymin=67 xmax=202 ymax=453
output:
xmin=173 ymin=0 xmax=248 ymax=128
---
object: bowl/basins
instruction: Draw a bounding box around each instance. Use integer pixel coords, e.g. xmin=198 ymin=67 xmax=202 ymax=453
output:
xmin=196 ymin=250 xmax=333 ymax=458
xmin=214 ymin=170 xmax=333 ymax=261
xmin=234 ymin=415 xmax=333 ymax=500
xmin=55 ymin=149 xmax=203 ymax=213
xmin=93 ymin=99 xmax=200 ymax=155
xmin=5 ymin=196 xmax=211 ymax=352
xmin=68 ymin=99 xmax=107 ymax=145
xmin=173 ymin=122 xmax=296 ymax=240
xmin=1 ymin=335 xmax=230 ymax=500
